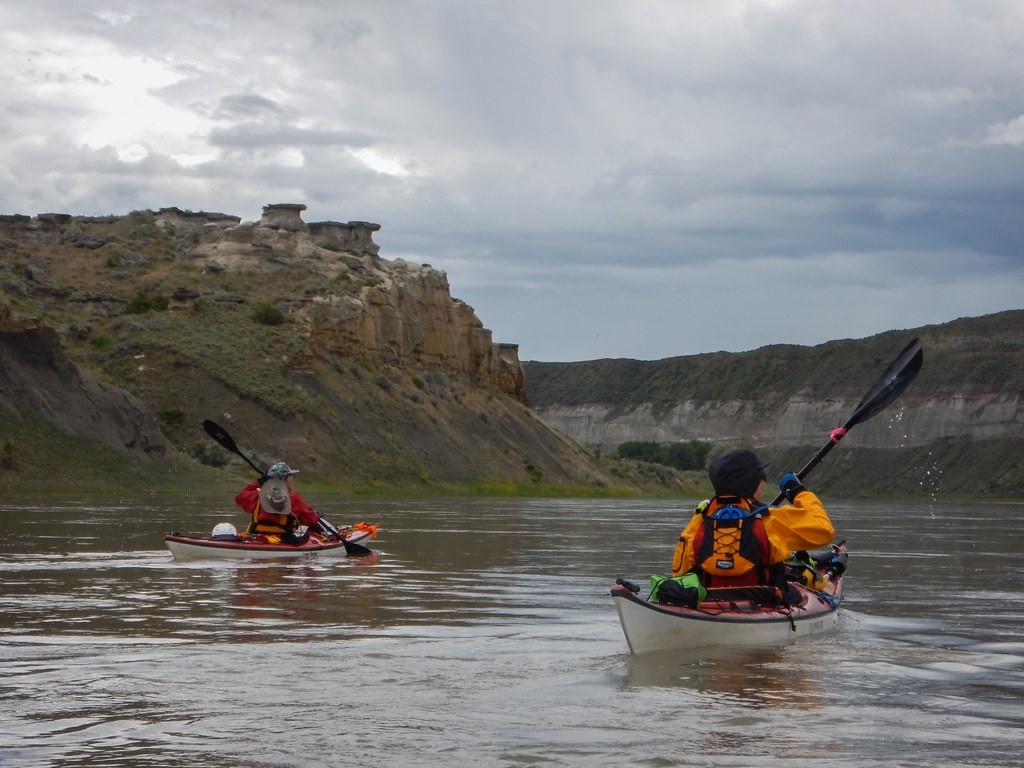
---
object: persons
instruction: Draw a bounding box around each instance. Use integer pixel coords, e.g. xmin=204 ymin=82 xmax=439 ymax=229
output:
xmin=234 ymin=462 xmax=324 ymax=535
xmin=671 ymin=448 xmax=836 ymax=589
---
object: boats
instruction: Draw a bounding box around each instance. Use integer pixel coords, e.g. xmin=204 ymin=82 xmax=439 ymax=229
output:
xmin=161 ymin=521 xmax=380 ymax=562
xmin=608 ymin=539 xmax=851 ymax=657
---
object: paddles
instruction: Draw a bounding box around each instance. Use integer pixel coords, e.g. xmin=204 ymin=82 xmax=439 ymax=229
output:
xmin=766 ymin=339 xmax=923 ymax=505
xmin=203 ymin=418 xmax=373 ymax=556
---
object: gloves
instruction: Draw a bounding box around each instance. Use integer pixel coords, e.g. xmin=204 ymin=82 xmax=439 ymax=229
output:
xmin=314 ymin=508 xmax=324 ymax=517
xmin=261 ymin=470 xmax=269 ymax=482
xmin=779 ymin=472 xmax=808 ymax=504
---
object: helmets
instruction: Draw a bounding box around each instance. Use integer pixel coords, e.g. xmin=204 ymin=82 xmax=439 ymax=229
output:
xmin=212 ymin=523 xmax=238 ymax=540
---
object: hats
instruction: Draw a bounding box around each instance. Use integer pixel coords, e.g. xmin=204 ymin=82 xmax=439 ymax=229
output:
xmin=708 ymin=448 xmax=769 ymax=493
xmin=267 ymin=462 xmax=299 ymax=477
xmin=259 ymin=478 xmax=291 ymax=515
